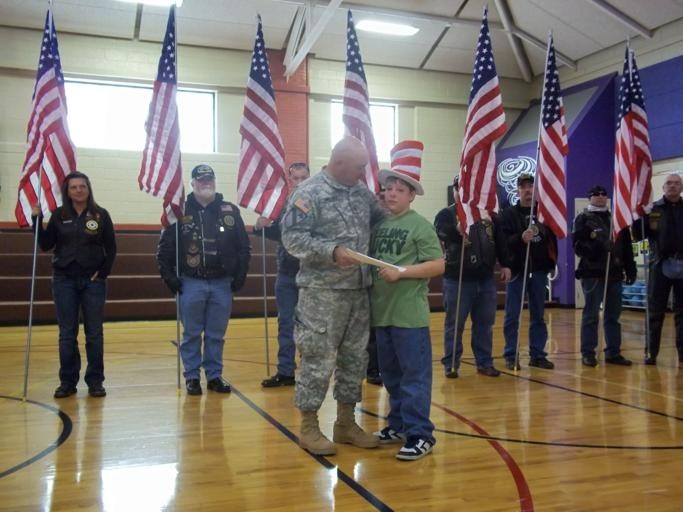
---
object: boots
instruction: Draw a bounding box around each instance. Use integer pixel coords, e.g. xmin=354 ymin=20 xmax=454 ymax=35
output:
xmin=333 ymin=401 xmax=379 ymax=448
xmin=301 ymin=411 xmax=337 ymax=455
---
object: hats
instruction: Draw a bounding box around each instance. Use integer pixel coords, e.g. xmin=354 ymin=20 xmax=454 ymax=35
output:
xmin=377 ymin=141 xmax=425 ymax=195
xmin=192 ymin=165 xmax=215 ymax=180
xmin=518 ymin=174 xmax=534 ymax=185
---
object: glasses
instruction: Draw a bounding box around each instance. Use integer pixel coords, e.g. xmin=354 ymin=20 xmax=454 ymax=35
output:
xmin=591 ymin=191 xmax=607 ymax=196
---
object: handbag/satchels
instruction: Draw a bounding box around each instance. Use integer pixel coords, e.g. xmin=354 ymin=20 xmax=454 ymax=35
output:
xmin=662 ymin=258 xmax=683 ymax=279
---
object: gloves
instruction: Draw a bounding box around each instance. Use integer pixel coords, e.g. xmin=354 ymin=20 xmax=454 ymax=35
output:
xmin=626 ymin=271 xmax=635 ymax=285
xmin=168 ymin=277 xmax=182 ymax=294
xmin=232 ymin=275 xmax=244 ymax=291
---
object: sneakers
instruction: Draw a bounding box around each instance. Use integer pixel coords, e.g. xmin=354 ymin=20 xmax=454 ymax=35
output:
xmin=395 ymin=438 xmax=432 ymax=460
xmin=89 ymin=384 xmax=106 ymax=397
xmin=54 ymin=384 xmax=77 ymax=397
xmin=445 ymin=357 xmax=554 ymax=378
xmin=372 ymin=426 xmax=405 ymax=443
xmin=582 ymin=355 xmax=656 ymax=367
xmin=262 ymin=374 xmax=295 ymax=387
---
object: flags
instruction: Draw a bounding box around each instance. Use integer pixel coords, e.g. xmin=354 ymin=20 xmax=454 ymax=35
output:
xmin=630 ymin=56 xmax=652 ymax=210
xmin=342 ymin=9 xmax=379 ymax=196
xmin=535 ymin=36 xmax=568 ymax=239
xmin=14 ymin=8 xmax=77 ymax=227
xmin=455 ymin=12 xmax=506 ymax=236
xmin=612 ymin=59 xmax=641 ymax=241
xmin=139 ymin=4 xmax=185 ymax=230
xmin=236 ymin=15 xmax=289 ymax=221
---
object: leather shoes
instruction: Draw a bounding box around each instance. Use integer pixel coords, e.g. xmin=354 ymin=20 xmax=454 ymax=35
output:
xmin=367 ymin=374 xmax=382 ymax=384
xmin=186 ymin=378 xmax=231 ymax=395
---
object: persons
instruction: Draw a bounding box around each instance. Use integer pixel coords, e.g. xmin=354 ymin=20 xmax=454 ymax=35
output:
xmin=280 ymin=136 xmax=386 ymax=456
xmin=365 ymin=141 xmax=445 ymax=461
xmin=572 ymin=186 xmax=638 ymax=366
xmin=32 ymin=172 xmax=116 ymax=398
xmin=434 ymin=176 xmax=512 ymax=378
xmin=157 ymin=165 xmax=251 ymax=395
xmin=501 ymin=174 xmax=558 ymax=370
xmin=633 ymin=174 xmax=683 ymax=365
xmin=367 ymin=328 xmax=382 ymax=385
xmin=253 ymin=164 xmax=311 ymax=386
